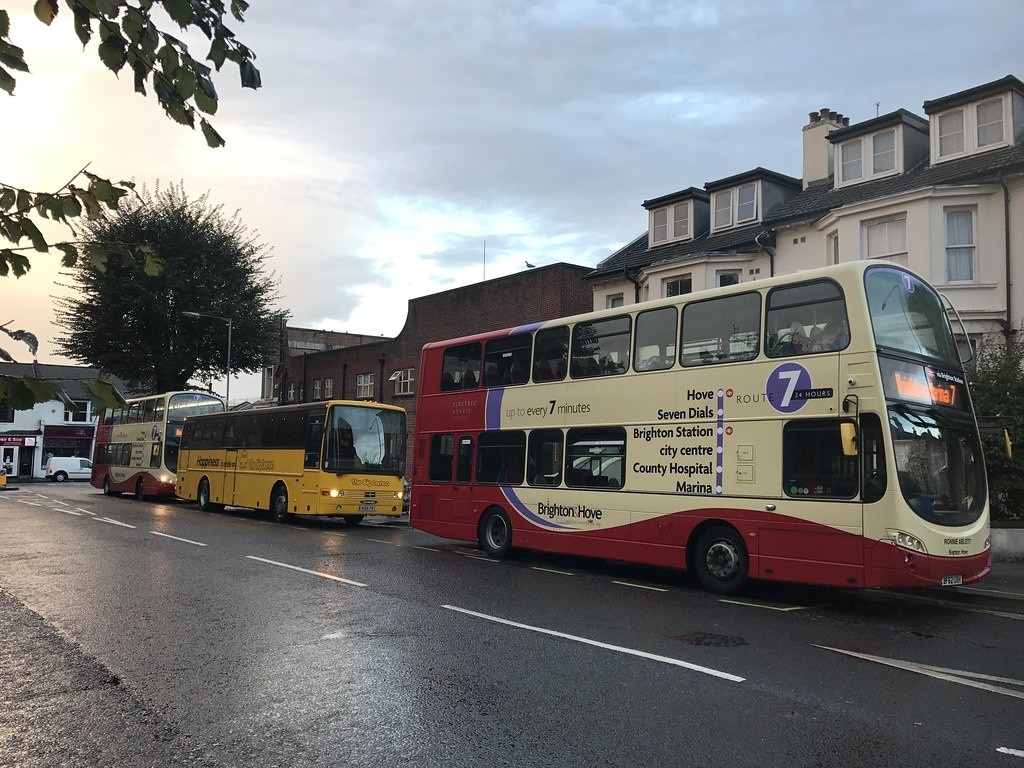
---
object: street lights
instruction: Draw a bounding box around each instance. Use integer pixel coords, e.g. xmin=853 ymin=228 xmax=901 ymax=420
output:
xmin=181 ymin=311 xmax=232 ymax=411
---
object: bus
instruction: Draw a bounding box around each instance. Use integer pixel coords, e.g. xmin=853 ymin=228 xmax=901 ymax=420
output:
xmin=176 ymin=400 xmax=408 ymax=527
xmin=407 ymin=258 xmax=1013 ymax=595
xmin=90 ymin=391 xmax=226 ymax=502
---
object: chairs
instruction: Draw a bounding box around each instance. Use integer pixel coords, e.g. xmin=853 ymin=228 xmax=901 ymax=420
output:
xmin=430 ymin=454 xmax=620 ymax=487
xmin=441 ymin=323 xmax=822 ymax=391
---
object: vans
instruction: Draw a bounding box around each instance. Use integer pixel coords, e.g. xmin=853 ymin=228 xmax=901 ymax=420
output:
xmin=45 ymin=457 xmax=92 ymax=482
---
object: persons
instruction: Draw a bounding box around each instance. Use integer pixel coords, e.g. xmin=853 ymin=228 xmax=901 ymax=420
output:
xmin=810 ymin=319 xmax=848 ymax=354
